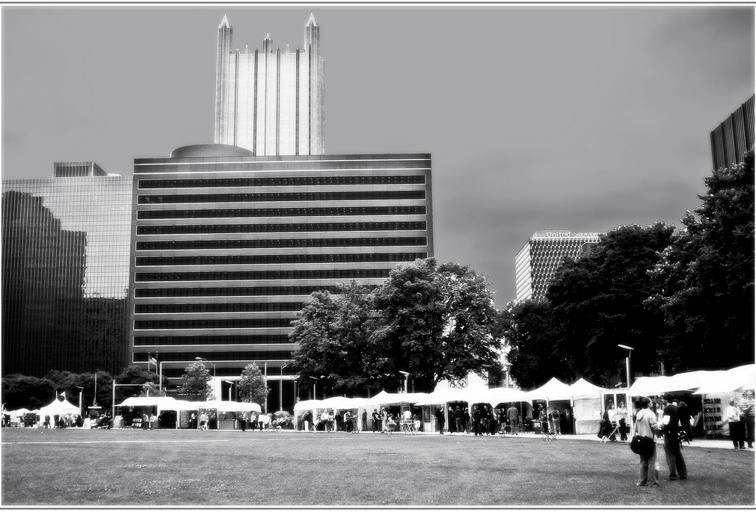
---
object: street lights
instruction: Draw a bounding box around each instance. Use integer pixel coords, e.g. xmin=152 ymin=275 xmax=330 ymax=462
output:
xmin=195 ymin=356 xmax=216 ymax=399
xmin=618 ymin=343 xmax=635 ymax=436
xmin=399 ymin=369 xmax=410 ymax=391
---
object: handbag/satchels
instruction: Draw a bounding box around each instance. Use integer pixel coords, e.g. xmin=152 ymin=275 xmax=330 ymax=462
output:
xmin=629 ymin=435 xmax=656 ymax=457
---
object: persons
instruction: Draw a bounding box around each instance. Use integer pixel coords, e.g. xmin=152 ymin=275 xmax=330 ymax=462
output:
xmin=719 ymin=389 xmax=755 ymax=450
xmin=657 ymin=392 xmax=687 ymax=481
xmin=598 ymin=388 xmax=702 ymax=448
xmin=635 ymin=397 xmax=663 ymax=487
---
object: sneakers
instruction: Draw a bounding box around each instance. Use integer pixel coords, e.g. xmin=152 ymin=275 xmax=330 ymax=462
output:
xmin=663 ymin=474 xmax=688 ymax=481
xmin=637 ymin=480 xmax=660 ymax=488
